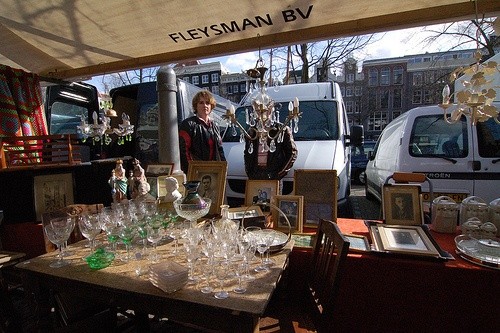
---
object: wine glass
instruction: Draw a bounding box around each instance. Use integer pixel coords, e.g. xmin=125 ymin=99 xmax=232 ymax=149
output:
xmin=78 ymin=199 xmax=180 ymax=277
xmin=45 ymin=217 xmax=76 ymax=269
xmin=165 ymin=197 xmax=275 ymax=299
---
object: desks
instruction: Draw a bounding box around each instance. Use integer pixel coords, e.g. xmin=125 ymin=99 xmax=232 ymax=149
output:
xmin=12 ymin=215 xmax=294 ymax=333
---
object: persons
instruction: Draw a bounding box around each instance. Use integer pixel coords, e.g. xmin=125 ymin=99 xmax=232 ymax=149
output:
xmin=282 ymin=203 xmax=294 ymax=225
xmin=199 ymin=175 xmax=215 ymax=202
xmin=111 ymin=157 xmax=155 ymax=200
xmin=165 ymin=177 xmax=181 ymax=202
xmin=254 ymin=189 xmax=269 ymax=211
xmin=178 ymin=90 xmax=227 ymax=174
xmin=244 ymin=97 xmax=298 ymax=181
xmin=396 ymin=194 xmax=411 ymax=243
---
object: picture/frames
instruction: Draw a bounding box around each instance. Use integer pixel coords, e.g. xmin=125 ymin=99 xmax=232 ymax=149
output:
xmin=244 ymin=170 xmax=337 ymax=235
xmin=375 ymin=185 xmax=440 ymax=257
xmin=146 ymin=160 xmax=228 ymax=217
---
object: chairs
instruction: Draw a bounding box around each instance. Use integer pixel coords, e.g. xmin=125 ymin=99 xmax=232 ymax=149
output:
xmin=42 ymin=203 xmax=104 ymax=255
xmin=385 ymin=172 xmax=434 ymax=225
xmin=301 ymin=217 xmax=351 ymax=333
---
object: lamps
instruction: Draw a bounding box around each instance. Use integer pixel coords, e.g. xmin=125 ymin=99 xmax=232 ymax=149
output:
xmin=220 ymin=33 xmax=304 ymax=155
xmin=438 ymin=0 xmax=500 ymax=126
xmin=77 ymin=74 xmax=134 ymax=145
